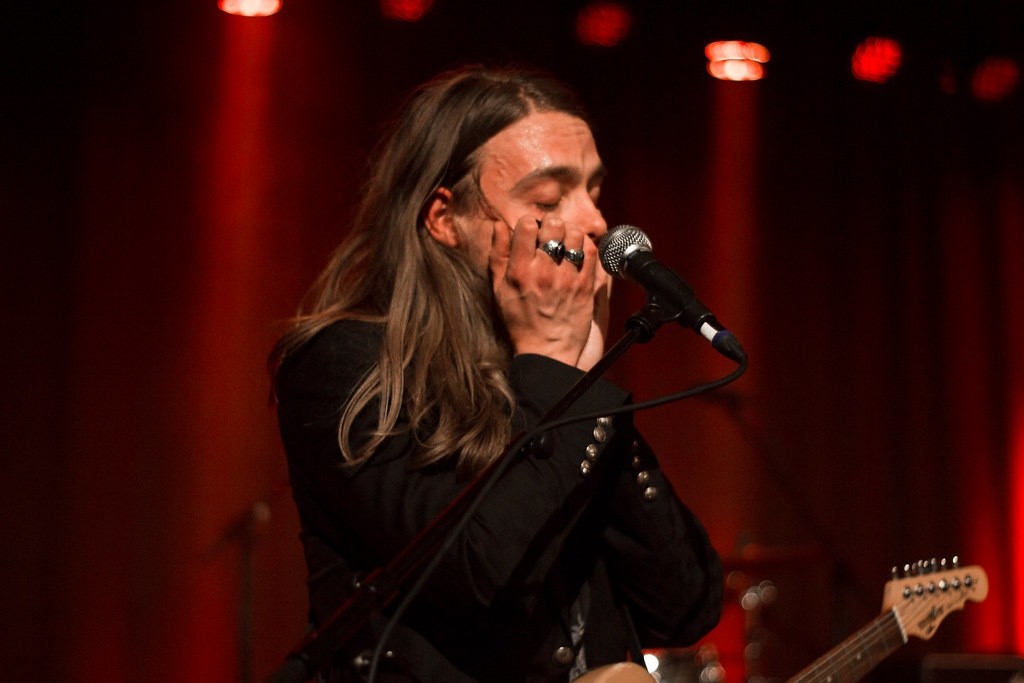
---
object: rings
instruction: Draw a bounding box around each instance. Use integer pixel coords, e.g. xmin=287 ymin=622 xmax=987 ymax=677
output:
xmin=565 ymin=249 xmax=584 ymax=266
xmin=536 ymin=238 xmax=565 ymax=262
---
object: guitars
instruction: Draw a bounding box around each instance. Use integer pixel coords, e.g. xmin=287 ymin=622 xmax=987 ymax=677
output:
xmin=568 ymin=555 xmax=990 ymax=683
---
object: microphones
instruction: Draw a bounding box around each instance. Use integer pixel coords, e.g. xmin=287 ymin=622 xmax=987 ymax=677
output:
xmin=600 ymin=225 xmax=746 ymax=363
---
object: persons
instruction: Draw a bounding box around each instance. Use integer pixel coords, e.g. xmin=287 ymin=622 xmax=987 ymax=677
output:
xmin=265 ymin=66 xmax=725 ymax=683
